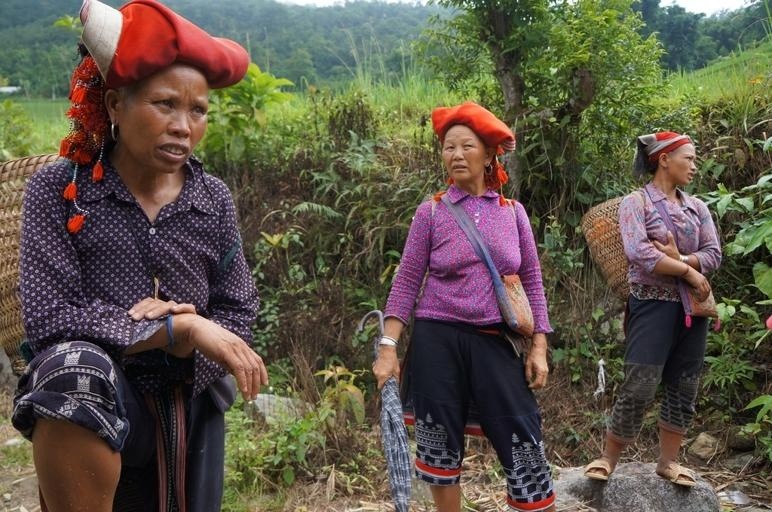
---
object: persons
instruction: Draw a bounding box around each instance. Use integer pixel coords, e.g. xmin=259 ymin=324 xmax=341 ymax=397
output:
xmin=368 ymin=98 xmax=563 ymax=512
xmin=9 ymin=1 xmax=271 ymax=512
xmin=581 ymin=131 xmax=723 ymax=490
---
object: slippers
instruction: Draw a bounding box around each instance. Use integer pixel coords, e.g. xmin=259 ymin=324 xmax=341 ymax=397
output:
xmin=584 ymin=459 xmax=612 ymax=480
xmin=664 ymin=463 xmax=696 ymax=486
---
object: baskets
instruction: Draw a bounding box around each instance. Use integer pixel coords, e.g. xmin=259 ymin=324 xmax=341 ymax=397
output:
xmin=579 ymin=196 xmax=633 ymax=302
xmin=0 ymin=153 xmax=71 ymax=376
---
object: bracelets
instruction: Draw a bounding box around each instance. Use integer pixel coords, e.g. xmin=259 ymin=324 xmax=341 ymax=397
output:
xmin=165 ymin=314 xmax=176 ymax=348
xmin=679 ymin=264 xmax=691 ymax=278
xmin=379 ymin=336 xmax=399 ymax=348
xmin=679 ymin=255 xmax=691 ymax=263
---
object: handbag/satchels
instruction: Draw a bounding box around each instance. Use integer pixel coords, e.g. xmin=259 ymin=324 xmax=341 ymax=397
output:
xmin=496 ymin=274 xmax=534 ymax=339
xmin=678 ymin=274 xmax=719 ymax=318
